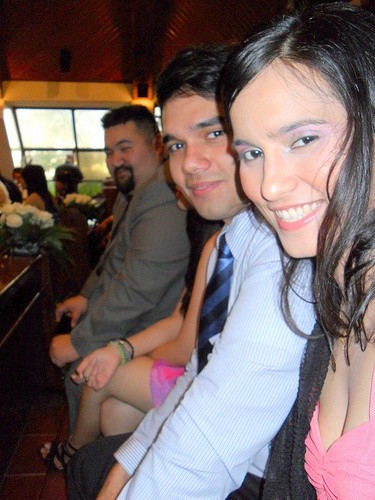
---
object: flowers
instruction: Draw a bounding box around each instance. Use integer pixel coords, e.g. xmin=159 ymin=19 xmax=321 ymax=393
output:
xmin=0 ymin=202 xmax=55 ymax=229
xmin=62 ymin=193 xmax=103 ymax=211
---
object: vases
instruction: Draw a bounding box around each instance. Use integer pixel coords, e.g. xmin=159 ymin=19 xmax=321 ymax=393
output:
xmin=11 ymin=242 xmax=44 ymax=257
xmin=65 ymin=207 xmax=81 ymax=219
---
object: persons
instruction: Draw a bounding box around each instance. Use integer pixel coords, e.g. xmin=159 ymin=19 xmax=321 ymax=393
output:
xmin=222 ymin=0 xmax=375 ymax=500
xmin=39 ymin=185 xmax=221 ymax=472
xmin=0 ymin=154 xmax=84 ymax=206
xmin=64 ymin=39 xmax=317 ymax=500
xmin=49 ymin=104 xmax=190 ymax=437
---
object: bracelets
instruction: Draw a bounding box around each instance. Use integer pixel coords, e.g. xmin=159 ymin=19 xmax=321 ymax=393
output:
xmin=109 ymin=337 xmax=135 ymax=365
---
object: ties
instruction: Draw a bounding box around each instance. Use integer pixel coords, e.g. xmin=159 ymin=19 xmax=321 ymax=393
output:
xmin=197 ymin=231 xmax=234 ymax=374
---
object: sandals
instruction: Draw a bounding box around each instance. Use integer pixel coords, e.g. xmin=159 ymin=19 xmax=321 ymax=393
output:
xmin=40 ymin=437 xmax=77 ymax=473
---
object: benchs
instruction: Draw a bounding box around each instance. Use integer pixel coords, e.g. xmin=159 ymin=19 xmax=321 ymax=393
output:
xmin=0 ymin=193 xmax=105 ymax=440
